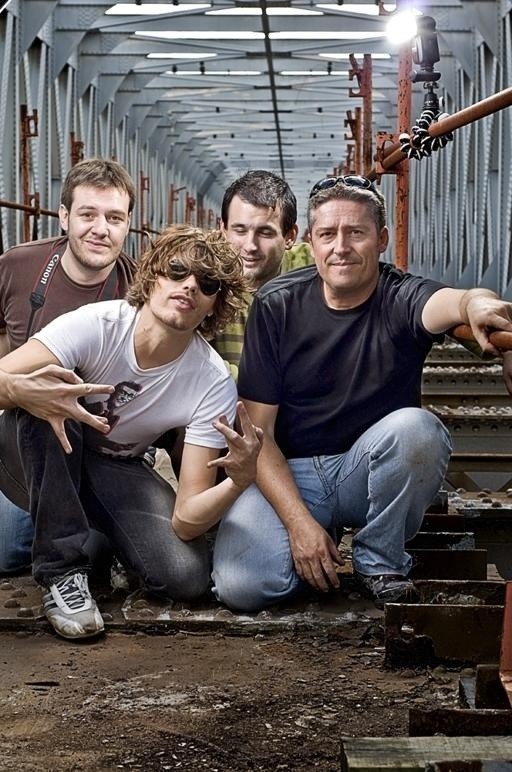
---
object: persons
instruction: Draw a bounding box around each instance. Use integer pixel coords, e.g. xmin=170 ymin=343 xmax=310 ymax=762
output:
xmin=167 ymin=169 xmax=315 ymax=557
xmin=0 ymin=160 xmax=147 ymax=581
xmin=210 ymin=173 xmax=512 ymax=617
xmin=0 ymin=222 xmax=268 ymax=642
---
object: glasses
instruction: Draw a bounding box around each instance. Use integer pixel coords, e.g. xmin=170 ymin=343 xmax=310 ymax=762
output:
xmin=155 ymin=256 xmax=222 ymax=297
xmin=310 ymin=174 xmax=379 ymax=199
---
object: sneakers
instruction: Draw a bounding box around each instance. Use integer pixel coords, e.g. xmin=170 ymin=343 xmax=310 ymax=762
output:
xmin=353 ymin=567 xmax=420 ymax=609
xmin=40 ymin=573 xmax=105 ymax=639
